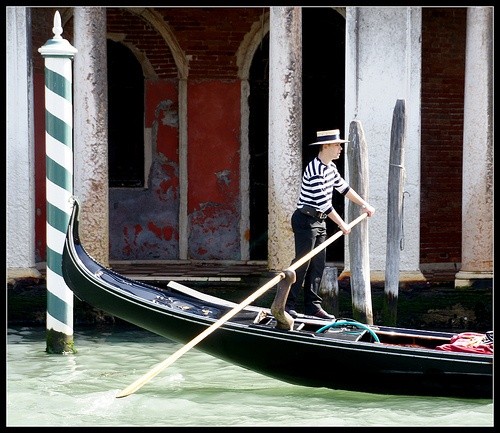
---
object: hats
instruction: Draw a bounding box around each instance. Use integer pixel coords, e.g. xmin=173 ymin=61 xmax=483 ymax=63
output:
xmin=308 ymin=128 xmax=351 ymax=145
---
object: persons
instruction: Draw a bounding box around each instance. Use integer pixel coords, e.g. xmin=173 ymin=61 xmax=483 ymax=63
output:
xmin=283 ymin=129 xmax=377 ymax=320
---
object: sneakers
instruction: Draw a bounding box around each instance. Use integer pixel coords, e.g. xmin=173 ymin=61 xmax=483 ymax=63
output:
xmin=315 ymin=307 xmax=335 ymax=320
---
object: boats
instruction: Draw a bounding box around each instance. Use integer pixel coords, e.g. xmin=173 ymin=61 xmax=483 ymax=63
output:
xmin=60 ymin=200 xmax=494 ymax=402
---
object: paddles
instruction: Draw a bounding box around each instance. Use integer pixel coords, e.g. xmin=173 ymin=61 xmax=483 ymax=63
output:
xmin=114 ymin=211 xmax=371 ymax=399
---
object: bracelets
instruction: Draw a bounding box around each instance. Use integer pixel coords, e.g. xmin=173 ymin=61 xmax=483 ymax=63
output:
xmin=337 ymin=220 xmax=345 ymax=228
xmin=360 ymin=202 xmax=370 ymax=209
